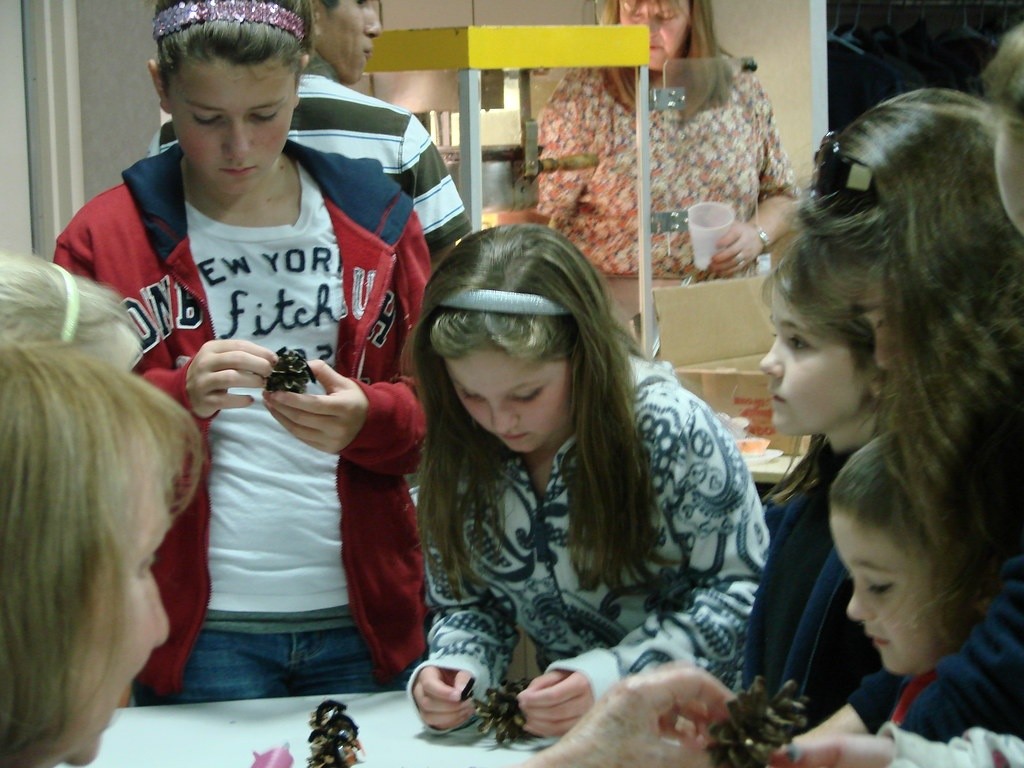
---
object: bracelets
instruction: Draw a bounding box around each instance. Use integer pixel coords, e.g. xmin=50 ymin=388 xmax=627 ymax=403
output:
xmin=751 ymin=224 xmax=769 ymax=252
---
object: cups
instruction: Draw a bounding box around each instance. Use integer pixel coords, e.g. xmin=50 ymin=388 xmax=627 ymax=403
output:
xmin=687 ymin=202 xmax=734 ymax=269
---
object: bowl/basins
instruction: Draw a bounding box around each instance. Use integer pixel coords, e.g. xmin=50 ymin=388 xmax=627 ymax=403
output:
xmin=737 ymin=438 xmax=771 ymax=455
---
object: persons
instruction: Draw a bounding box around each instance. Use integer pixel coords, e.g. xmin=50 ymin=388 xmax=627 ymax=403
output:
xmin=405 ymin=224 xmax=769 ymax=735
xmin=0 ymin=256 xmax=140 ymax=374
xmin=1 ymin=341 xmax=733 ymax=768
xmin=55 ymin=0 xmax=432 ymax=706
xmin=742 ymin=29 xmax=1024 ymax=768
xmin=537 ymin=0 xmax=803 ymax=283
xmin=150 ymin=0 xmax=471 ymax=272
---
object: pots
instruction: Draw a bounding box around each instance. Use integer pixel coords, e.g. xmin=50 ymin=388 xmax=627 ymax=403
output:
xmin=438 ymin=145 xmax=599 ymax=211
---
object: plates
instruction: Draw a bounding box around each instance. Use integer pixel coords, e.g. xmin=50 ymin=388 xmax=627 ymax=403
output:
xmin=743 ymin=450 xmax=784 ymax=465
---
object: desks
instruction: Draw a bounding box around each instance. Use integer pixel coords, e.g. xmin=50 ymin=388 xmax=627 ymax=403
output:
xmin=50 ymin=685 xmax=578 ymax=768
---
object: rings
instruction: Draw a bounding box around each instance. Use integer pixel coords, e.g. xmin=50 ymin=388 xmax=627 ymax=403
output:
xmin=738 ymin=254 xmax=743 ymax=262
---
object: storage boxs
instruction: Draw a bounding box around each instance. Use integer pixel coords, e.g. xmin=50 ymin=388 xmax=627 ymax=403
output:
xmin=673 ymin=346 xmax=813 ymax=454
xmin=343 ymin=24 xmax=650 ymax=362
